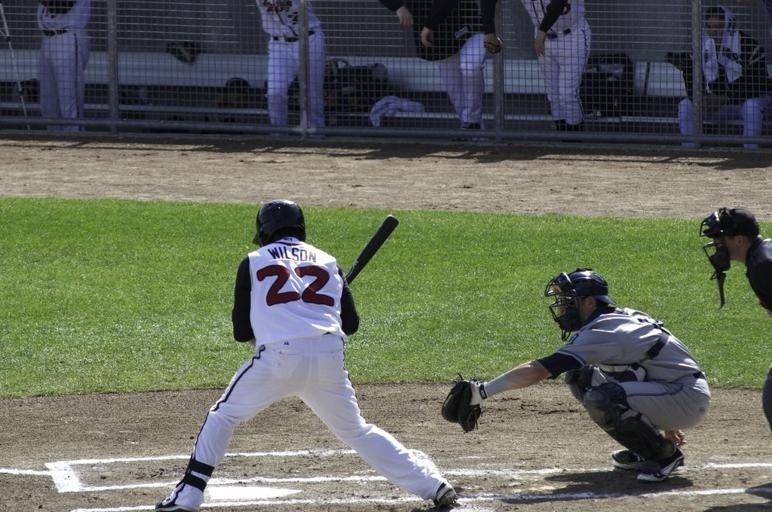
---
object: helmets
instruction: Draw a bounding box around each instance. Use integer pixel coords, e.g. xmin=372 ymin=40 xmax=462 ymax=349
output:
xmin=699 ymin=207 xmax=761 ymax=272
xmin=545 ymin=267 xmax=613 ymax=340
xmin=254 ymin=200 xmax=305 ymax=246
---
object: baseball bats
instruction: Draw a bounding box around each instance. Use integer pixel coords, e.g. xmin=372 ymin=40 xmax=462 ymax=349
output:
xmin=345 ymin=214 xmax=400 ymax=285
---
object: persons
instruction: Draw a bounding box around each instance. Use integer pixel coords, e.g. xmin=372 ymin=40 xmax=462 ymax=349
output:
xmin=443 ymin=264 xmax=714 ymax=483
xmin=254 ymin=1 xmax=331 ymax=140
xmin=696 ymin=203 xmax=772 ymax=432
xmin=33 ymin=1 xmax=97 ymax=137
xmin=381 ymin=1 xmax=496 ymax=141
xmin=148 ymin=194 xmax=466 ymax=510
xmin=662 ymin=6 xmax=772 ymax=154
xmin=477 ymin=1 xmax=595 ymax=144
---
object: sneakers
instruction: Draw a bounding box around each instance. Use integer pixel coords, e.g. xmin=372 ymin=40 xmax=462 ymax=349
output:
xmin=434 ymin=481 xmax=457 ymax=507
xmin=612 ymin=450 xmax=683 ymax=482
xmin=154 ymin=497 xmax=193 ymax=512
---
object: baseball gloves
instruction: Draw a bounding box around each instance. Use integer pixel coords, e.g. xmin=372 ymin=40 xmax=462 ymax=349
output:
xmin=440 ymin=372 xmax=482 ymax=433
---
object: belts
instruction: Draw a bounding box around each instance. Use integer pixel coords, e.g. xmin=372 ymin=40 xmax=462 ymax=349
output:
xmin=546 ymin=28 xmax=570 ymax=39
xmin=42 ymin=28 xmax=68 ymax=36
xmin=274 ymin=28 xmax=314 ymax=43
